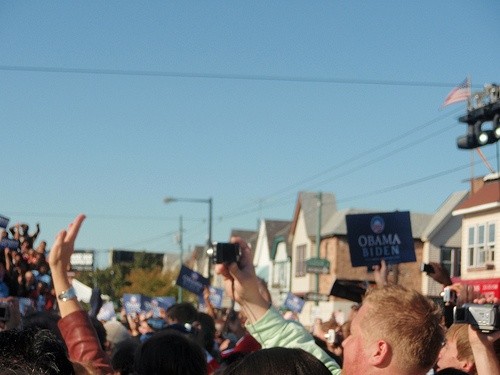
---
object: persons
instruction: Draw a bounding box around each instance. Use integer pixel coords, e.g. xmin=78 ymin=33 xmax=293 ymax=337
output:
xmin=0 ymin=215 xmax=500 ymax=375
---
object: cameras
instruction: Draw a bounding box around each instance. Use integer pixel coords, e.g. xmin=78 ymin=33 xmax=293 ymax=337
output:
xmin=421 ymin=264 xmax=434 ymax=274
xmin=440 ymin=288 xmax=456 ymax=304
xmin=207 ymin=242 xmax=240 ymax=264
xmin=324 ymin=329 xmax=339 ymax=346
xmin=453 ymin=303 xmax=500 ymax=333
xmin=0 ymin=301 xmax=11 ymax=323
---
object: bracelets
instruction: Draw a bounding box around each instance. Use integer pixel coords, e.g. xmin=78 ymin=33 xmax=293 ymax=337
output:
xmin=59 ymin=287 xmax=77 ymax=301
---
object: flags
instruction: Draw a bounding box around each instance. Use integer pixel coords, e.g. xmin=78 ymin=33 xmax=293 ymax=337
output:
xmin=443 ymin=78 xmax=468 ymax=106
